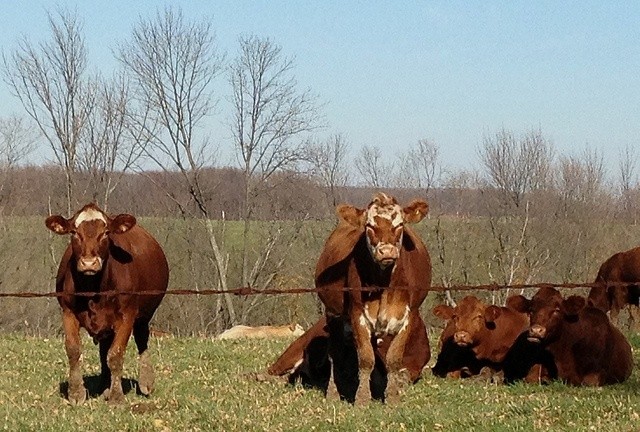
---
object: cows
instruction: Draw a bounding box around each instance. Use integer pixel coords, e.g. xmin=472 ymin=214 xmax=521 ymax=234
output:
xmin=489 ymin=286 xmax=634 ymax=388
xmin=425 ymin=295 xmax=530 ymax=380
xmin=44 ymin=202 xmax=169 ymax=408
xmin=586 ymin=247 xmax=640 ymax=327
xmin=315 ymin=191 xmax=431 ymax=409
xmin=256 ymin=310 xmax=430 ymax=390
